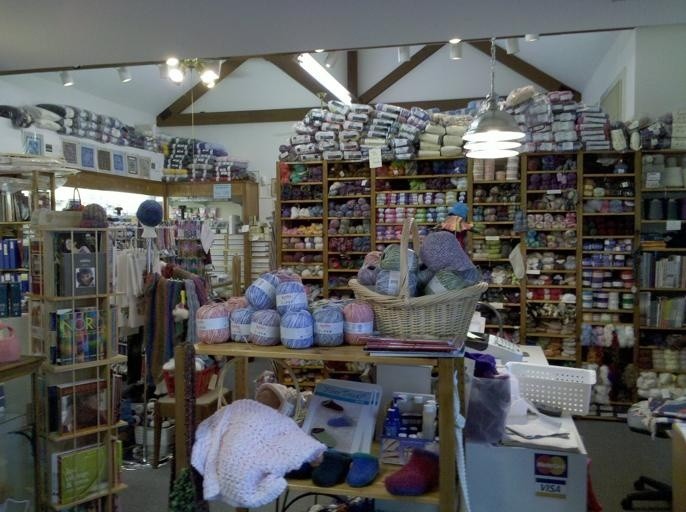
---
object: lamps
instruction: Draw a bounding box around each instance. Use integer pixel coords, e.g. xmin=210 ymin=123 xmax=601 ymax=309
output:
xmin=45 ymin=32 xmax=544 ymax=105
xmin=463 ymin=38 xmax=527 ymax=160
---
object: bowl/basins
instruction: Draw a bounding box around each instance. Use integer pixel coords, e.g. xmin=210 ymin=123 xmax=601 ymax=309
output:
xmin=49 ymin=211 xmax=83 ymax=228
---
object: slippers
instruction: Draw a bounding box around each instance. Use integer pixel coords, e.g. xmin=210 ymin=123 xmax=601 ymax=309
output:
xmin=384 ymin=448 xmax=440 ymax=496
xmin=312 ymin=449 xmax=351 ymax=487
xmin=347 ymin=452 xmax=380 ymax=487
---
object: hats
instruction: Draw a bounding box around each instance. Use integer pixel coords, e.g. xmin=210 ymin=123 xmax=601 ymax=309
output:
xmin=137 ymin=199 xmax=162 ymax=227
xmin=79 ymin=203 xmax=109 ymax=228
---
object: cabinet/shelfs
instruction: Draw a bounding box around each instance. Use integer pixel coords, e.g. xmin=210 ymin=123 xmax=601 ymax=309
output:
xmin=271 ymin=150 xmax=685 ymax=424
xmin=195 ymin=336 xmax=463 ymax=511
xmin=0 ymin=171 xmax=55 ymax=320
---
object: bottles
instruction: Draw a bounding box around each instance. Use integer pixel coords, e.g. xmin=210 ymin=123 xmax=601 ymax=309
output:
xmin=384 ymin=395 xmax=437 ymax=439
xmin=1 ymin=182 xmax=52 ymax=223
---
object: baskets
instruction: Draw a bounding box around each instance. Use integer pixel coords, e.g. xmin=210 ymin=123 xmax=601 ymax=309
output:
xmin=504 ymin=361 xmax=597 ymax=418
xmin=348 ymin=217 xmax=485 ymax=340
xmin=134 ymin=418 xmax=191 ymax=458
xmin=161 ymin=361 xmax=215 ymax=397
xmin=213 ymin=351 xmax=306 ymax=429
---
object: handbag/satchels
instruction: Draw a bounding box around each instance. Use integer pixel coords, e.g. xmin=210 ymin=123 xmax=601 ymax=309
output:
xmin=0 ymin=320 xmax=21 ymax=363
